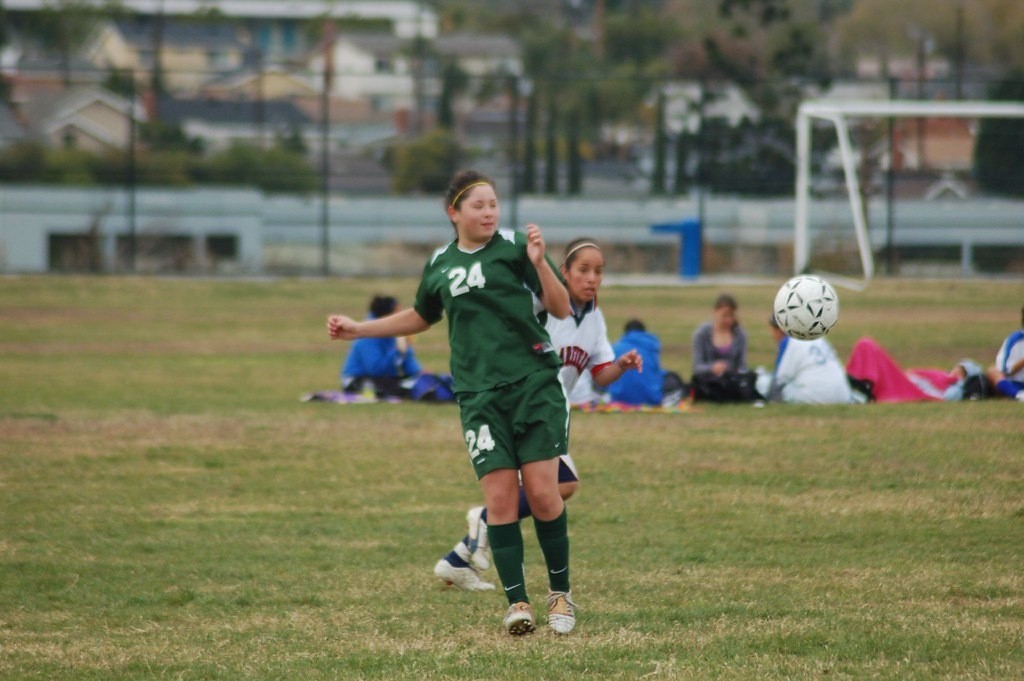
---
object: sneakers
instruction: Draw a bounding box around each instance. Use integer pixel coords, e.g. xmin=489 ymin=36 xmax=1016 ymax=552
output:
xmin=502 ymin=602 xmax=537 ymax=637
xmin=433 ymin=559 xmax=496 ymax=592
xmin=467 ymin=506 xmax=491 ymax=571
xmin=545 ymin=588 xmax=579 ymax=633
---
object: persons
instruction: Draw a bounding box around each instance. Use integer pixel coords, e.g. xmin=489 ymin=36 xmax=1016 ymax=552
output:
xmin=433 ymin=238 xmax=643 ymax=589
xmin=592 ymin=319 xmax=686 ymax=408
xmin=327 ymin=172 xmax=575 ymax=635
xmin=692 ymin=292 xmax=766 ymax=401
xmin=845 ymin=337 xmax=981 ymax=404
xmin=340 ymin=295 xmax=422 ymax=401
xmin=756 ymin=311 xmax=851 ymax=403
xmin=986 ymin=306 xmax=1024 ymax=400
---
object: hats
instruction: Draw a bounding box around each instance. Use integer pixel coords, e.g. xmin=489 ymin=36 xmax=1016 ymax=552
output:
xmin=959 ymin=360 xmax=980 ymax=377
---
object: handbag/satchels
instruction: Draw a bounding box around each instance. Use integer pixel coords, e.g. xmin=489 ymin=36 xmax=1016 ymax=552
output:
xmin=412 ymin=373 xmax=456 ymax=403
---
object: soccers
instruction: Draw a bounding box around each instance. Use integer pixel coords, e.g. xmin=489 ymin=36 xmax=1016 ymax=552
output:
xmin=772 ymin=274 xmax=839 ymax=341
